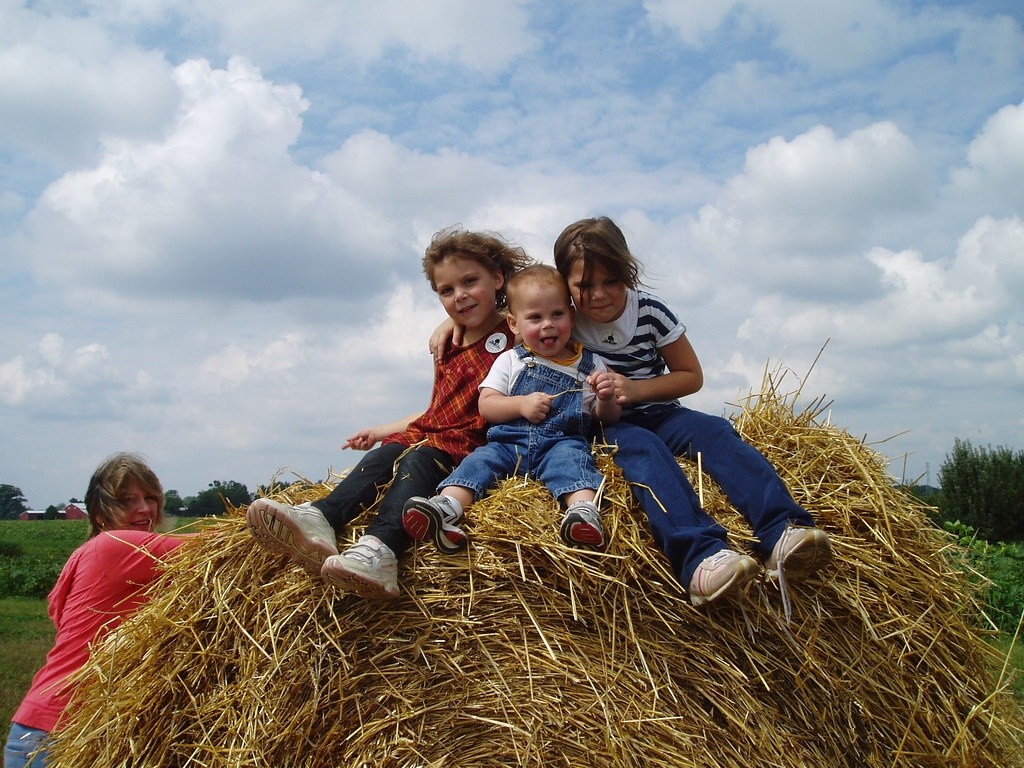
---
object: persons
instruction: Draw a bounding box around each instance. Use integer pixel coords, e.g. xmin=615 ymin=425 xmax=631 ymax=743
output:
xmin=3 ymin=451 xmax=218 ymax=768
xmin=403 ymin=264 xmax=623 ymax=553
xmin=247 ymin=223 xmax=536 ymax=600
xmin=430 ymin=217 xmax=832 ymax=606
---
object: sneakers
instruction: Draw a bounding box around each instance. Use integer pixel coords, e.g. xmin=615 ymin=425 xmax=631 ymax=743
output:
xmin=401 ymin=494 xmax=468 ymax=555
xmin=245 ymin=496 xmax=340 ymax=576
xmin=318 ymin=535 xmax=402 ymax=602
xmin=761 ymin=524 xmax=834 ymax=626
xmin=558 ymin=499 xmax=606 ymax=549
xmin=688 ymin=548 xmax=802 ymax=655
